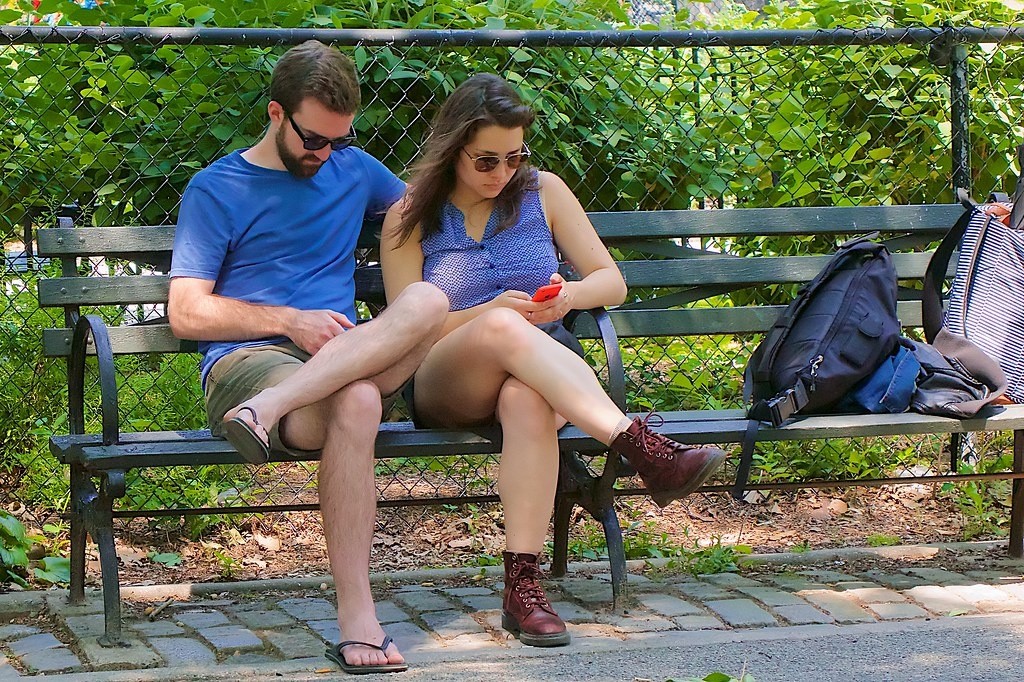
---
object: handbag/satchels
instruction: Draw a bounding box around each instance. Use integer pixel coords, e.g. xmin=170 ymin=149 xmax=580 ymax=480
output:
xmin=922 ymin=186 xmax=1024 ymax=405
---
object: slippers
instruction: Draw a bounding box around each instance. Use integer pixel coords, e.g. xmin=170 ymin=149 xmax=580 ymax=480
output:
xmin=219 ymin=407 xmax=271 ymax=464
xmin=325 ymin=635 xmax=408 ymax=674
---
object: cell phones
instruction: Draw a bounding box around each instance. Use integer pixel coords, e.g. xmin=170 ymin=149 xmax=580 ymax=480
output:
xmin=532 ymin=284 xmax=563 ymax=302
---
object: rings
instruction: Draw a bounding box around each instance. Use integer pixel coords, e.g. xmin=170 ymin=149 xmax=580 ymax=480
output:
xmin=564 ymin=292 xmax=567 ymax=297
xmin=529 ymin=312 xmax=531 ymax=320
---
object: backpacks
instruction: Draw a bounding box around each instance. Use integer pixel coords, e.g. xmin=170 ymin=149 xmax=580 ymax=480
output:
xmin=743 ymin=229 xmax=900 ymax=427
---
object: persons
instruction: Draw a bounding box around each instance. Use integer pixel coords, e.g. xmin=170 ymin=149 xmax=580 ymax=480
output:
xmin=379 ymin=77 xmax=728 ymax=646
xmin=167 ymin=38 xmax=449 ymax=672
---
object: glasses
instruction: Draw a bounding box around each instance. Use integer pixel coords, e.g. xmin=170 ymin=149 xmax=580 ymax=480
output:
xmin=271 ymin=98 xmax=358 ymax=152
xmin=461 ymin=140 xmax=532 ymax=172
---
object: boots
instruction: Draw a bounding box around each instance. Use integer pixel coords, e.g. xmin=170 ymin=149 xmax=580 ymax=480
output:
xmin=609 ymin=407 xmax=726 ymax=508
xmin=501 ymin=550 xmax=570 ymax=646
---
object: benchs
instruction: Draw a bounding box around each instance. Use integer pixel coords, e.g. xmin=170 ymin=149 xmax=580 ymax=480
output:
xmin=35 ymin=188 xmax=1024 ymax=650
